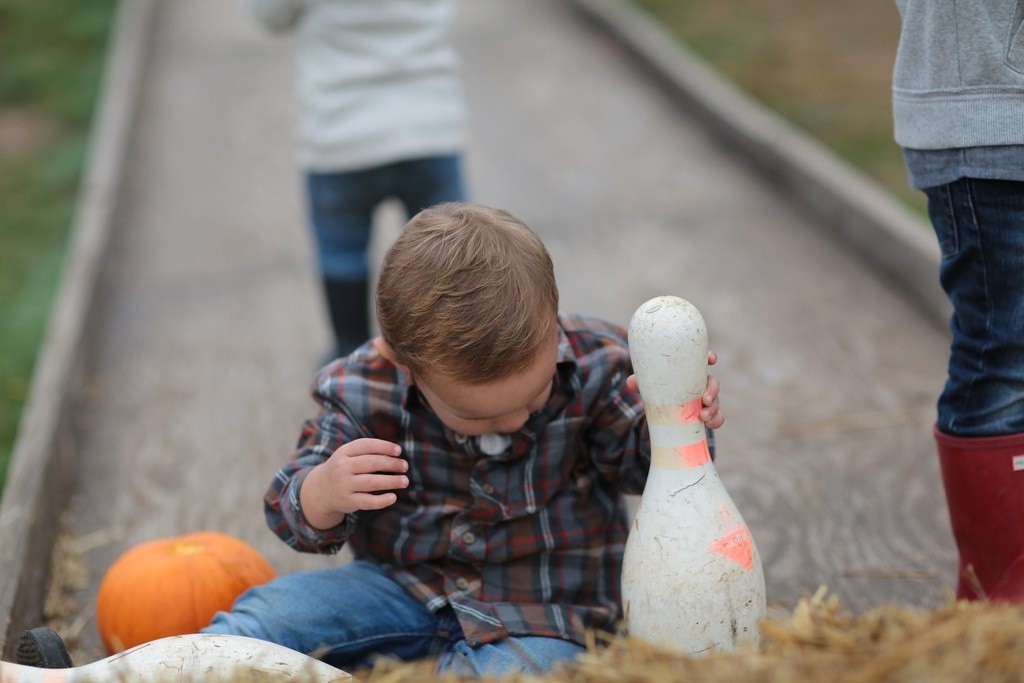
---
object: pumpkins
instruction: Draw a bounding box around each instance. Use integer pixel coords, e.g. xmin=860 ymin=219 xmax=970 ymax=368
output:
xmin=97 ymin=531 xmax=276 ymax=656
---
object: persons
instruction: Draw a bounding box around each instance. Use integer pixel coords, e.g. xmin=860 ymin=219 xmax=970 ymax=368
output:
xmin=254 ymin=0 xmax=474 ymax=371
xmin=18 ymin=197 xmax=723 ymax=678
xmin=892 ymin=0 xmax=1024 ymax=612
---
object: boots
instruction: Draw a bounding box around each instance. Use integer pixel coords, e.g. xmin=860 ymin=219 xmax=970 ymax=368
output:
xmin=322 ymin=276 xmax=371 ymax=364
xmin=932 ymin=423 xmax=1024 ymax=603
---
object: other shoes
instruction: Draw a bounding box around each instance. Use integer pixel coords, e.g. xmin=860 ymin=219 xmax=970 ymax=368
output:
xmin=16 ymin=627 xmax=73 ymax=669
